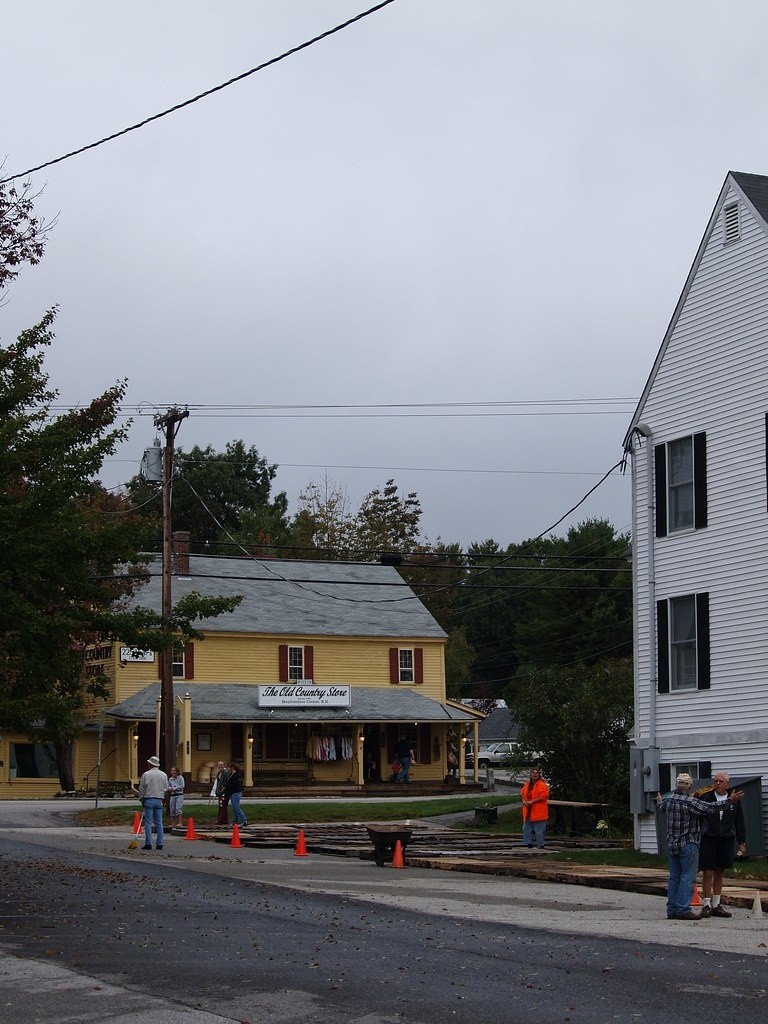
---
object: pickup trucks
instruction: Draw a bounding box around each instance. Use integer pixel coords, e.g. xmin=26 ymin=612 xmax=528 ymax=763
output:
xmin=464 ymin=742 xmax=555 ymax=770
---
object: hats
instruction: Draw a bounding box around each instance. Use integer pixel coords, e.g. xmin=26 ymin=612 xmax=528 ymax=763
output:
xmin=147 ymin=756 xmax=160 ymax=766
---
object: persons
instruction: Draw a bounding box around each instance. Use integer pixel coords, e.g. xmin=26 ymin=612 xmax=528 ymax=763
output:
xmin=447 ymin=735 xmax=460 ymax=776
xmin=657 ymin=774 xmax=745 ymax=920
xmin=699 ymin=772 xmax=746 ymax=918
xmin=139 ymin=756 xmax=168 ymax=850
xmin=167 ymin=766 xmax=184 ymax=827
xmin=522 ymin=769 xmax=548 ymax=848
xmin=210 ymin=761 xmax=247 ymax=826
xmin=392 ymin=735 xmax=414 ymax=784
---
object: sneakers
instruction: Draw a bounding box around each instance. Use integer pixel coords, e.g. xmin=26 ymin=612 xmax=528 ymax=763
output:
xmin=701 ymin=905 xmax=712 ymax=918
xmin=712 ymin=904 xmax=731 ymax=917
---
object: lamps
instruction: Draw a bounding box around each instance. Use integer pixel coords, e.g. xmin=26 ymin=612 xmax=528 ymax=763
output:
xmin=359 ymin=733 xmax=365 ymax=742
xmin=461 ymin=735 xmax=467 ymax=743
xmin=133 ymin=733 xmax=139 ymax=740
xmin=248 ymin=735 xmax=255 ymax=743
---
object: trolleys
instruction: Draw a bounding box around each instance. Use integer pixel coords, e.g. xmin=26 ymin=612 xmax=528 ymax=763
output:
xmin=365 ymin=824 xmax=424 ymax=867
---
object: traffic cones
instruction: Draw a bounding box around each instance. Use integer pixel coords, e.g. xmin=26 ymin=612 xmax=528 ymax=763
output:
xmin=132 ymin=812 xmax=143 ymax=834
xmin=183 ymin=818 xmax=197 ymax=840
xmin=390 ymin=839 xmax=405 ymax=869
xmin=294 ymin=829 xmax=309 ymax=856
xmin=228 ymin=824 xmax=243 ymax=848
xmin=750 ymin=891 xmax=766 ymax=919
xmin=689 ymin=882 xmax=703 ymax=906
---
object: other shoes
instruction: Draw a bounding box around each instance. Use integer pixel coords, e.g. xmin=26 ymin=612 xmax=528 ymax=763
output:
xmin=142 ymin=845 xmax=152 ymax=850
xmin=242 ymin=823 xmax=247 ymax=826
xmin=156 ymin=845 xmax=162 ymax=849
xmin=676 ymin=912 xmax=701 ymax=919
xmin=668 ymin=915 xmax=676 ymax=919
